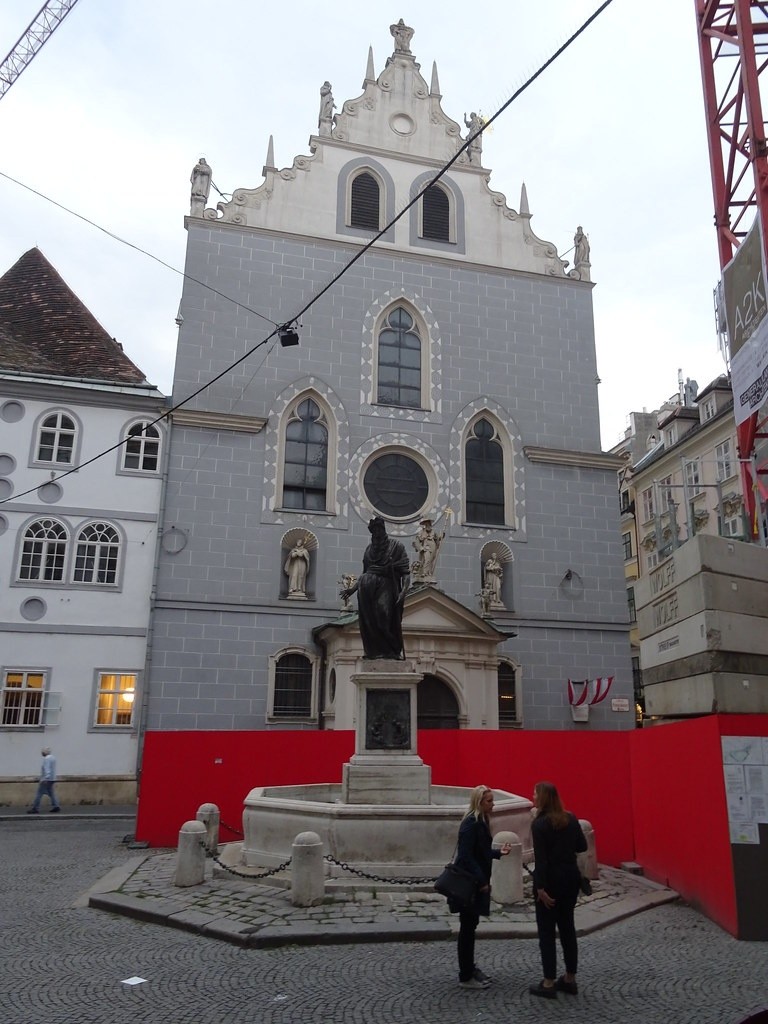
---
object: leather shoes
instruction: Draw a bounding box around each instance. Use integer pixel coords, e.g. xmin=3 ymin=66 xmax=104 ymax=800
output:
xmin=529 ymin=980 xmax=556 ymax=998
xmin=556 ymin=974 xmax=578 ymax=994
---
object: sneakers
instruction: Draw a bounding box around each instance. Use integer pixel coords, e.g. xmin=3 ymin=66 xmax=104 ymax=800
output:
xmin=459 ymin=977 xmax=491 ymax=988
xmin=473 ymin=968 xmax=493 ymax=981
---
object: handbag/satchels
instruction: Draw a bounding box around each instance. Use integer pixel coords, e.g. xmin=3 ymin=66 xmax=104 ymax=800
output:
xmin=580 ymin=876 xmax=592 ymax=896
xmin=434 ymin=863 xmax=478 ymax=907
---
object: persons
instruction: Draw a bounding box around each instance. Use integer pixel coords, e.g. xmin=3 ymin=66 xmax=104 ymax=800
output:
xmin=412 ymin=518 xmax=445 ymax=578
xmin=284 ymin=536 xmax=311 ymax=595
xmin=318 ymin=81 xmax=337 ymax=128
xmin=339 ymin=574 xmax=353 ymax=607
xmin=338 ymin=517 xmax=412 ymax=662
xmin=463 ymin=110 xmax=485 ymax=151
xmin=446 ymin=785 xmax=511 ymax=990
xmin=474 ymin=584 xmax=496 ymax=618
xmin=573 ymin=225 xmax=592 ymax=267
xmin=189 ymin=157 xmax=212 ymax=200
xmin=484 ymin=552 xmax=504 ymax=604
xmin=528 ymin=782 xmax=590 ymax=1000
xmin=27 ymin=745 xmax=61 ymax=814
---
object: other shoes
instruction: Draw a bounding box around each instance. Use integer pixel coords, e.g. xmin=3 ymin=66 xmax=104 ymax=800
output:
xmin=49 ymin=807 xmax=61 ymax=812
xmin=27 ymin=809 xmax=39 ymax=814
